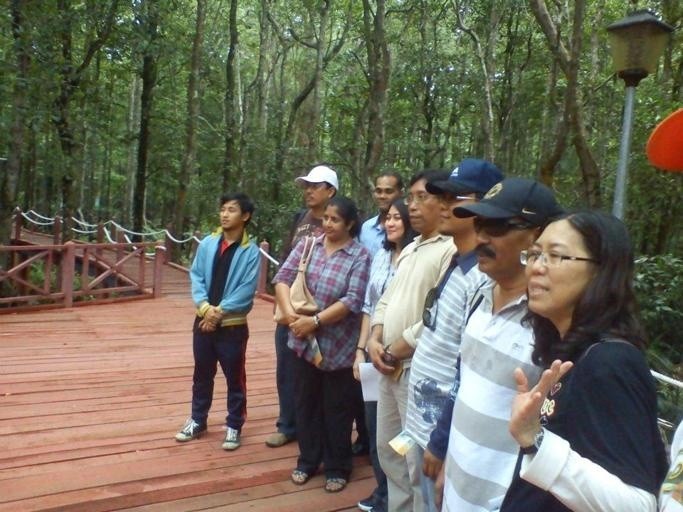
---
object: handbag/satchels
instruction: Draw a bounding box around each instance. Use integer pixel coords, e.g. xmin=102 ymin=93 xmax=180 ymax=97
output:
xmin=271 ymin=235 xmax=318 ymax=320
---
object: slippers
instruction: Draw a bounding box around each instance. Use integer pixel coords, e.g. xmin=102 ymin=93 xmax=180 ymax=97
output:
xmin=292 ymin=458 xmax=317 ymax=485
xmin=325 ymin=468 xmax=351 ymax=492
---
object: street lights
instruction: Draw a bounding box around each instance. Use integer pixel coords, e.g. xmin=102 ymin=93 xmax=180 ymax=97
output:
xmin=601 ymin=5 xmax=675 ymax=228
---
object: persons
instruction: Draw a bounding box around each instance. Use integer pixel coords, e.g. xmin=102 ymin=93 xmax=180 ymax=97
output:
xmin=175 ymin=192 xmax=263 ymax=450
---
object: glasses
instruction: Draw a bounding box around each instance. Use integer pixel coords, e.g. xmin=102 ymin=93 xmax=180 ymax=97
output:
xmin=423 ymin=286 xmax=441 ymax=332
xmin=401 ymin=192 xmax=444 ymax=206
xmin=464 ymin=216 xmax=536 ymax=236
xmin=518 ymin=246 xmax=598 ymax=272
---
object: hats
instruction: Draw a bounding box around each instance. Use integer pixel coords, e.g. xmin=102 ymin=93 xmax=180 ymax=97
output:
xmin=453 ymin=176 xmax=563 ymax=222
xmin=295 ymin=163 xmax=341 ymax=192
xmin=426 ymin=158 xmax=507 ymax=192
xmin=646 ymin=100 xmax=683 ymax=174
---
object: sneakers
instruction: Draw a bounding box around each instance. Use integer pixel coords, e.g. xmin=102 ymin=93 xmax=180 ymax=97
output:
xmin=222 ymin=419 xmax=243 ymax=453
xmin=348 ymin=435 xmax=371 ymax=456
xmin=175 ymin=415 xmax=210 ymax=444
xmin=357 ymin=485 xmax=389 ymax=511
xmin=264 ymin=422 xmax=296 ymax=448
